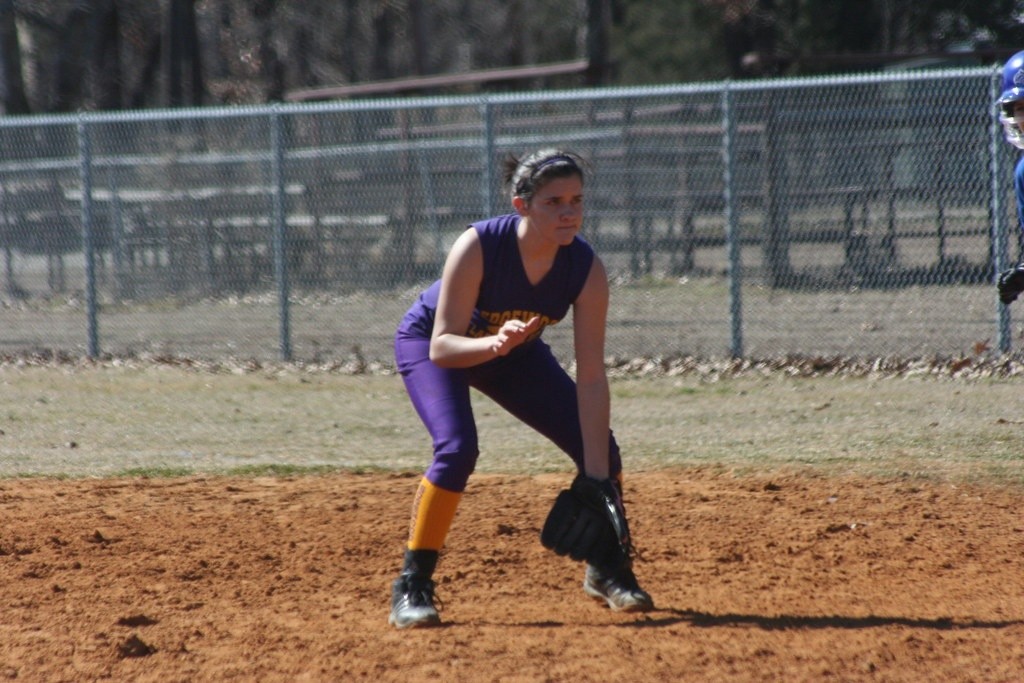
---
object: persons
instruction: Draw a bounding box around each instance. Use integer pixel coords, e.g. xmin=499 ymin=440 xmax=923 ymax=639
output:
xmin=994 ymin=51 xmax=1024 ymax=306
xmin=388 ymin=148 xmax=653 ymax=629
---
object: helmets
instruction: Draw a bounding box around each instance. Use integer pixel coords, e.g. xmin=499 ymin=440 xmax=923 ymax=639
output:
xmin=994 ymin=50 xmax=1024 ymax=150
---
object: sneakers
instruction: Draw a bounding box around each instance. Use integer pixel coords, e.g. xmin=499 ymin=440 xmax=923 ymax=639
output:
xmin=584 ymin=561 xmax=653 ymax=611
xmin=389 ymin=571 xmax=443 ymax=628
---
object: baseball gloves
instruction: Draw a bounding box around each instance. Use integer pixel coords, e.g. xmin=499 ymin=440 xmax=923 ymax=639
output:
xmin=538 ymin=477 xmax=630 ymax=567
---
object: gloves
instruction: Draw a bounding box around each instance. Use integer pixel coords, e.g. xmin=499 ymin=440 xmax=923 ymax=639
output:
xmin=997 ymin=268 xmax=1024 ymax=304
